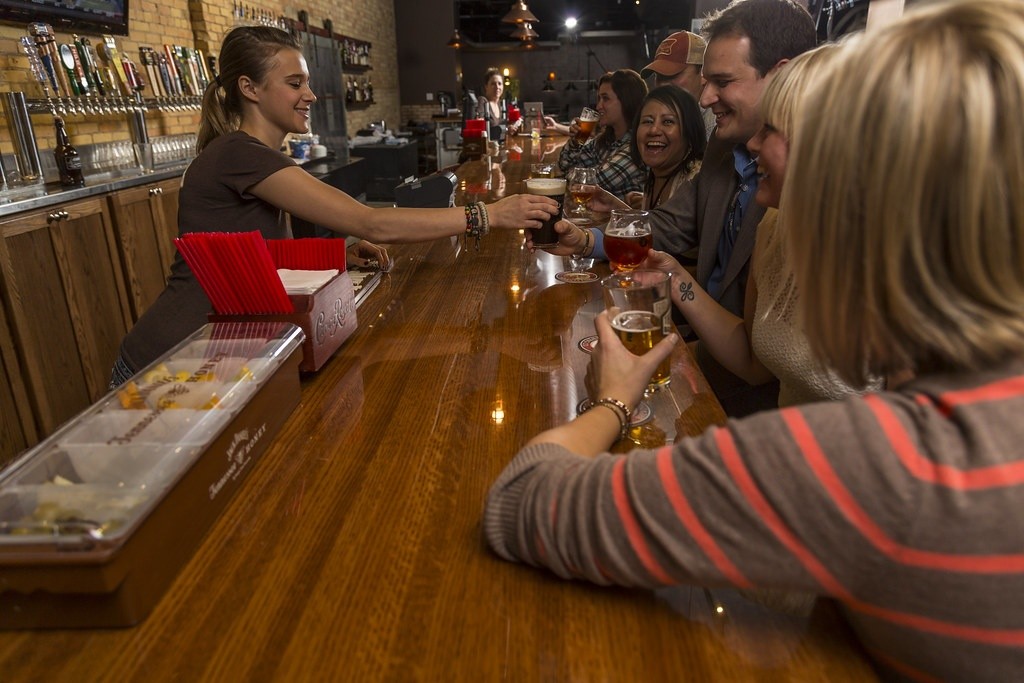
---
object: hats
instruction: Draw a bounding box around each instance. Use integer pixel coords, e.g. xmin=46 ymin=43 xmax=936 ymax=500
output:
xmin=641 ymin=30 xmax=708 ymax=79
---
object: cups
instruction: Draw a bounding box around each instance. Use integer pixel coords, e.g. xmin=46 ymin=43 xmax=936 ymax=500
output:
xmin=572 ymin=107 xmax=601 ymax=146
xmin=526 ymin=179 xmax=567 ymax=248
xmin=601 ymin=268 xmax=672 ymax=398
xmin=531 ymin=163 xmax=552 ymax=179
xmin=89 ymin=141 xmax=135 ymax=168
xmin=148 ymin=132 xmax=198 ymax=164
xmin=531 ymin=120 xmax=540 ymax=138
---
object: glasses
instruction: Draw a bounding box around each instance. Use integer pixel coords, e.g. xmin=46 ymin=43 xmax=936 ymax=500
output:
xmin=726 ymin=186 xmax=743 ymax=249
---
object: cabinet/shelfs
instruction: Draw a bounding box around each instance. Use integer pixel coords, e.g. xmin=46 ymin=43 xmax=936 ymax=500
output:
xmin=0 ymin=177 xmax=293 ymax=476
xmin=343 ymin=63 xmax=376 ymax=106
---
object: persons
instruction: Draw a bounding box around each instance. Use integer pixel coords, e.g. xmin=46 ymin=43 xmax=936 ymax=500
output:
xmin=484 ymin=0 xmax=1023 ymax=683
xmin=571 ymin=83 xmax=706 ymax=341
xmin=110 ymin=25 xmax=559 ymax=391
xmin=474 ymin=67 xmax=518 ymax=140
xmin=639 ymin=30 xmax=716 ymax=141
xmin=524 ymin=0 xmax=819 ymax=419
xmin=558 ymin=67 xmax=649 ymax=203
xmin=609 ymin=46 xmax=916 ymax=408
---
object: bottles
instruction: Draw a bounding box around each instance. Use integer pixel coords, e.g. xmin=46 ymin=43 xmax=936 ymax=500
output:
xmin=338 ymin=39 xmax=369 ymax=65
xmin=346 ymin=73 xmax=373 ymax=104
xmin=502 ymin=98 xmax=521 ymax=120
xmin=54 ymin=117 xmax=86 ymax=192
xmin=380 ymin=119 xmax=387 ymax=134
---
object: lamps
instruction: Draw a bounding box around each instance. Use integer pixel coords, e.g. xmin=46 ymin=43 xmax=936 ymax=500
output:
xmin=501 ymin=0 xmax=541 ymax=49
xmin=540 ymin=82 xmax=599 ymax=93
xmin=447 ymin=29 xmax=466 ymax=50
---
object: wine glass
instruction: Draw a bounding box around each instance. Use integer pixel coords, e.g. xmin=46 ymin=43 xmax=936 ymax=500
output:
xmin=601 ymin=209 xmax=654 ymax=289
xmin=569 ymin=167 xmax=597 ymax=214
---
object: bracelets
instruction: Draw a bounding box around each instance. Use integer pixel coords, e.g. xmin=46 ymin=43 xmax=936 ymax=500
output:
xmin=462 ymin=201 xmax=489 ymax=252
xmin=585 ymin=402 xmax=625 ymax=441
xmin=572 ymin=227 xmax=589 ymax=261
xmin=583 ymin=397 xmax=631 ymax=438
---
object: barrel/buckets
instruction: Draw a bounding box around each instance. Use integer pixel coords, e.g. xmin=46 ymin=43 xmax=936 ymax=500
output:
xmin=288 ymin=140 xmax=312 ymax=159
